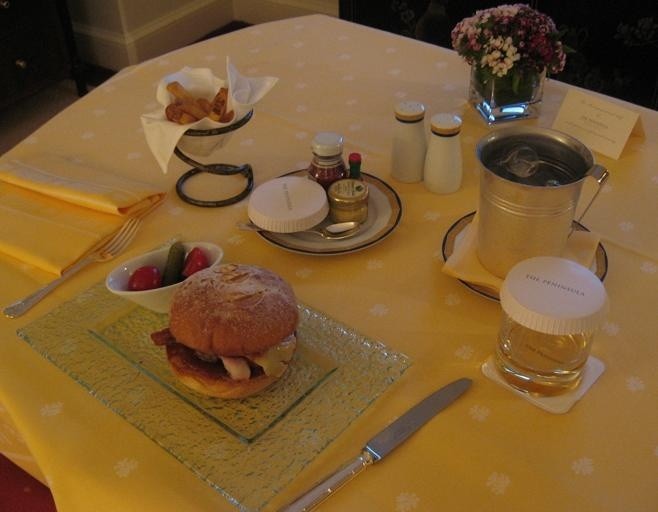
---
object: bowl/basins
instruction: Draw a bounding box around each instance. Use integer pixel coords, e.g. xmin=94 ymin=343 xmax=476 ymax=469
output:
xmin=106 ymin=239 xmax=216 ymax=318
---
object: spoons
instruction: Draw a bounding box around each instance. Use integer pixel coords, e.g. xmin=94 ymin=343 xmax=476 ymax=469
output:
xmin=242 ymin=221 xmax=360 ymax=242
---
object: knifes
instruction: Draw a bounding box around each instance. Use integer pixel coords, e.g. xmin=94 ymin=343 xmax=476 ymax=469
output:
xmin=272 ymin=374 xmax=472 ymax=511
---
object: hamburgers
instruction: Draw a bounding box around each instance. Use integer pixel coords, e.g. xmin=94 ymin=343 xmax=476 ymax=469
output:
xmin=151 ymin=263 xmax=299 ymax=400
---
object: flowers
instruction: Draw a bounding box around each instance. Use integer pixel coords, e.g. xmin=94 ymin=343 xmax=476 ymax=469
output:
xmin=447 ymin=0 xmax=565 ymax=107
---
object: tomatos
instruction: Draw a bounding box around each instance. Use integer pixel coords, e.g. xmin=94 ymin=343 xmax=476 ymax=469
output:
xmin=127 ymin=267 xmax=160 ymax=291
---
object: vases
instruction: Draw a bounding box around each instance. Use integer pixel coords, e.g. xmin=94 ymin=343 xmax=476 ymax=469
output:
xmin=466 ymin=64 xmax=544 ymax=118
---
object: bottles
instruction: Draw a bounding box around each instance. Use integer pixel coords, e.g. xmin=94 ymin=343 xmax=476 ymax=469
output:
xmin=342 ymin=149 xmax=367 ymax=182
xmin=328 ymin=178 xmax=368 ymax=223
xmin=424 ymin=111 xmax=463 ymax=198
xmin=492 ymin=256 xmax=608 ymax=400
xmin=389 ymin=97 xmax=426 ymax=185
xmin=304 ymin=129 xmax=349 ymax=185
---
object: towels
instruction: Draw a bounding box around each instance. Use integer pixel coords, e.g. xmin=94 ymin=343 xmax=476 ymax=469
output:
xmin=2 ymin=153 xmax=167 ymax=274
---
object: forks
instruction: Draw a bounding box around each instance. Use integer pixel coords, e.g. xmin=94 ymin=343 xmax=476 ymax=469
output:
xmin=2 ymin=215 xmax=141 ymax=321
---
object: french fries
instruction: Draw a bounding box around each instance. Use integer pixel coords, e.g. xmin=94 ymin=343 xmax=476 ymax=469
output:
xmin=166 ymin=82 xmax=228 ymax=124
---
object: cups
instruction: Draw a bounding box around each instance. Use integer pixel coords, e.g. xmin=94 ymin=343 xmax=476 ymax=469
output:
xmin=472 ymin=126 xmax=609 ymax=273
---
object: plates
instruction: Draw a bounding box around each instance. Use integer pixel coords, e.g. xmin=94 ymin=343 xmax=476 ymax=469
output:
xmin=257 ymin=167 xmax=403 ymax=257
xmin=14 ymin=260 xmax=415 ymax=509
xmin=440 ymin=208 xmax=608 ymax=301
